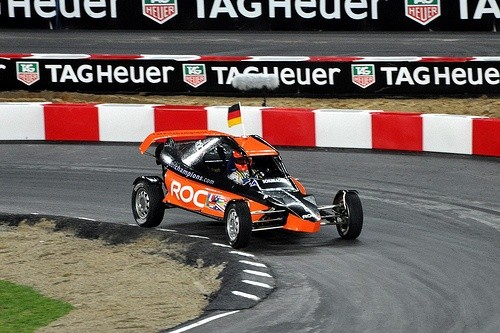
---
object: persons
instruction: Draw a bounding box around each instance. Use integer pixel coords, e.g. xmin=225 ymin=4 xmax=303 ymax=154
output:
xmin=230 ymin=148 xmax=265 ymax=188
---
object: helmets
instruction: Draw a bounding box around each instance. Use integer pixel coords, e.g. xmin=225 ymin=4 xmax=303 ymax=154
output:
xmin=233 ymin=151 xmax=252 ymax=171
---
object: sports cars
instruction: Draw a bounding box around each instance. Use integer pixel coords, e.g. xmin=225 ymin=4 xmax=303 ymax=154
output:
xmin=131 ymin=130 xmax=364 ymax=249
